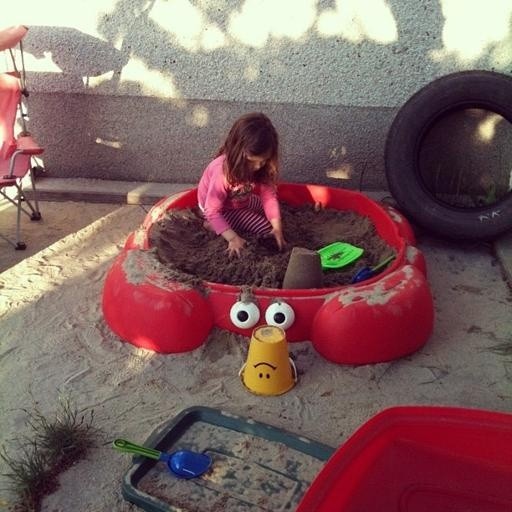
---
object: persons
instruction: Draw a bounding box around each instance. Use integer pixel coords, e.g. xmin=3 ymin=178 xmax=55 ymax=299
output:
xmin=195 ymin=112 xmax=293 ymax=260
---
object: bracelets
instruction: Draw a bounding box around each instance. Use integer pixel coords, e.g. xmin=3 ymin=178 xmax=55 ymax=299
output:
xmin=228 ymin=234 xmax=239 ymax=242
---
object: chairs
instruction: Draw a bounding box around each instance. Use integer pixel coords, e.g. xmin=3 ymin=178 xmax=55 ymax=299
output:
xmin=2 ymin=22 xmax=48 ymax=251
